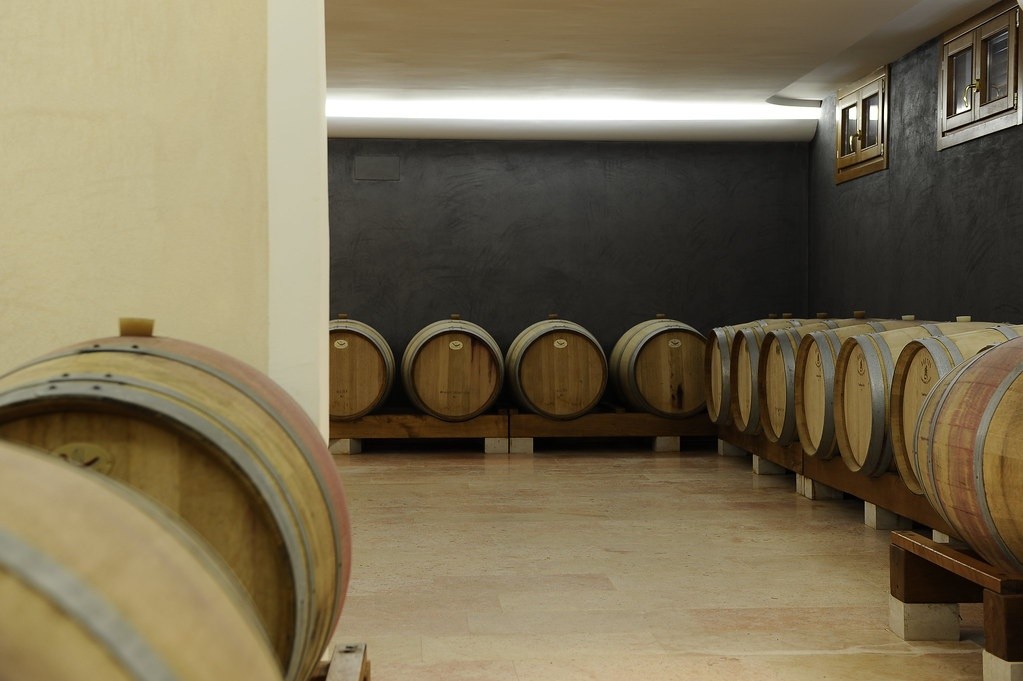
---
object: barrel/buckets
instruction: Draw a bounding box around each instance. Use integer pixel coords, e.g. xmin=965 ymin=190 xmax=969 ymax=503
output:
xmin=0 ymin=304 xmax=1023 ymax=681
